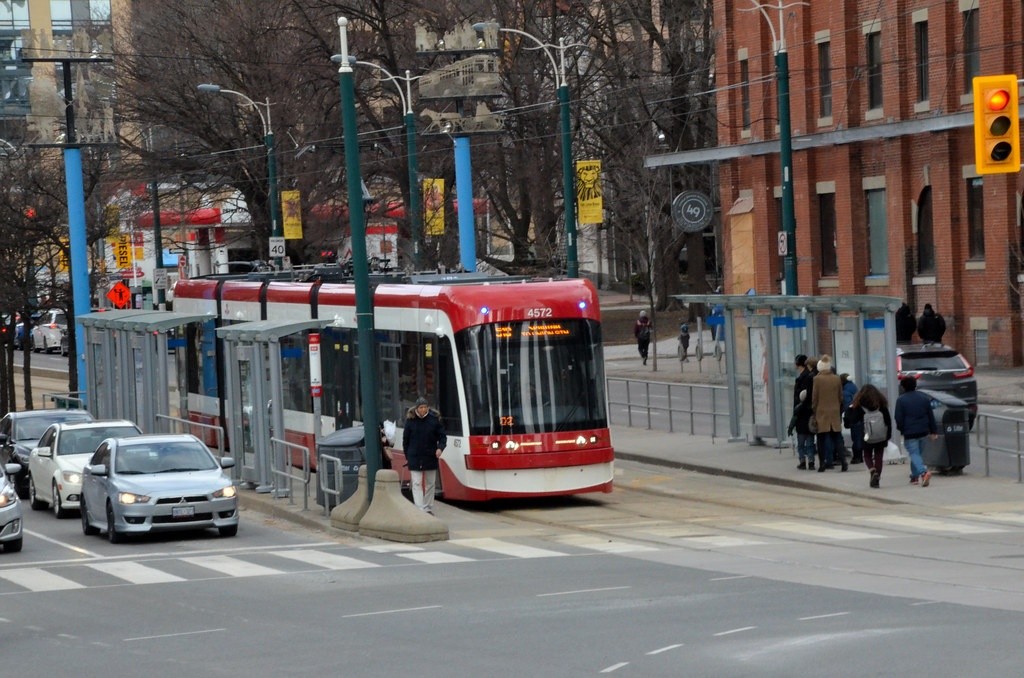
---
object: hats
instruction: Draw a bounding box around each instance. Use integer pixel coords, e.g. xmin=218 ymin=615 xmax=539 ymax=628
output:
xmin=681 ymin=325 xmax=688 ymax=333
xmin=840 ymin=373 xmax=852 ymax=385
xmin=416 ymin=396 xmax=428 ymax=407
xmin=640 ymin=311 xmax=647 ymax=317
xmin=800 ymin=389 xmax=808 ymax=401
xmin=817 ymin=354 xmax=832 ymax=372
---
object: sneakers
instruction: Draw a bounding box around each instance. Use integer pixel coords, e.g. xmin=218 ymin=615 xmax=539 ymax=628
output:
xmin=910 ymin=469 xmax=931 ymax=487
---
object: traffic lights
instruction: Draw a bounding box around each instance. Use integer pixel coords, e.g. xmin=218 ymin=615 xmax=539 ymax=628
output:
xmin=972 ymin=74 xmax=1022 ymax=175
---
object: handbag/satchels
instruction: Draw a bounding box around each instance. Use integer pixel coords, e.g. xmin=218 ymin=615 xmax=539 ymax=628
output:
xmin=808 ymin=417 xmax=818 ymax=433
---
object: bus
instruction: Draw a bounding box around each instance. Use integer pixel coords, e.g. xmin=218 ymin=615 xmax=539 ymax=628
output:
xmin=173 ymin=265 xmax=614 ymax=515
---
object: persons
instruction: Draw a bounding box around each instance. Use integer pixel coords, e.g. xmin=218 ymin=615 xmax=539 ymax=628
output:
xmin=892 ymin=302 xmax=916 ymax=345
xmin=400 ymin=397 xmax=447 ymax=517
xmin=678 ymin=324 xmax=691 ymax=363
xmin=633 ymin=309 xmax=654 ymax=366
xmin=787 ymin=352 xmax=865 ymax=474
xmin=844 ymin=383 xmax=893 ymax=488
xmin=917 ymin=302 xmax=946 ymax=346
xmin=895 ymin=376 xmax=938 ymax=487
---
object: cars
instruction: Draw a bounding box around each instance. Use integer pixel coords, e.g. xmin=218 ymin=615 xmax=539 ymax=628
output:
xmin=0 ymin=460 xmax=23 ymax=554
xmin=60 ymin=329 xmax=70 ymax=356
xmin=79 ymin=433 xmax=240 ymax=543
xmin=0 ymin=409 xmax=97 ymax=499
xmin=0 ymin=309 xmax=43 ymax=351
xmin=28 ymin=420 xmax=145 ymax=519
xmin=32 ymin=308 xmax=68 ymax=354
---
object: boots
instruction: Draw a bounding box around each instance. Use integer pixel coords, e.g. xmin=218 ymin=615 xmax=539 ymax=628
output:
xmin=869 ymin=467 xmax=879 ymax=488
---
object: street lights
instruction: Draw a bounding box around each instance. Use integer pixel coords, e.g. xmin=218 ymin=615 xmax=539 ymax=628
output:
xmin=198 ymin=81 xmax=286 ymax=271
xmin=328 ymin=50 xmax=435 ymax=273
xmin=471 ymin=19 xmax=593 ymax=276
xmin=735 ymin=0 xmax=801 ymax=297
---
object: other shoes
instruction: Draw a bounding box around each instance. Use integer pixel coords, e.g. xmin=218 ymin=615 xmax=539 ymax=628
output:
xmin=832 ymin=460 xmax=842 ymax=465
xmin=851 ymin=457 xmax=863 ymax=464
xmin=826 ymin=462 xmax=834 ymax=469
xmin=685 ymin=358 xmax=690 ymax=363
xmin=797 ymin=462 xmax=806 ymax=469
xmin=809 ymin=462 xmax=815 ymax=470
xmin=818 ymin=463 xmax=825 ymax=472
xmin=842 ymin=462 xmax=848 ymax=471
xmin=642 ymin=357 xmax=648 ymax=365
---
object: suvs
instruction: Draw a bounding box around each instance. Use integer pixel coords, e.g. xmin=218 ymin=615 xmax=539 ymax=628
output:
xmin=895 ymin=340 xmax=977 ymax=431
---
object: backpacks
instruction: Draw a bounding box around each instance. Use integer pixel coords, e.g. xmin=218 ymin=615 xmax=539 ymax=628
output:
xmin=863 ymin=410 xmax=888 ymax=443
xmin=639 ymin=326 xmax=650 ymax=341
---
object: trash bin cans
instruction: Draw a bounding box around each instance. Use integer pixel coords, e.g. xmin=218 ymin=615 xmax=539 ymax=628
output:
xmin=314 ymin=425 xmax=365 ymax=510
xmin=915 ymin=389 xmax=971 ymax=476
